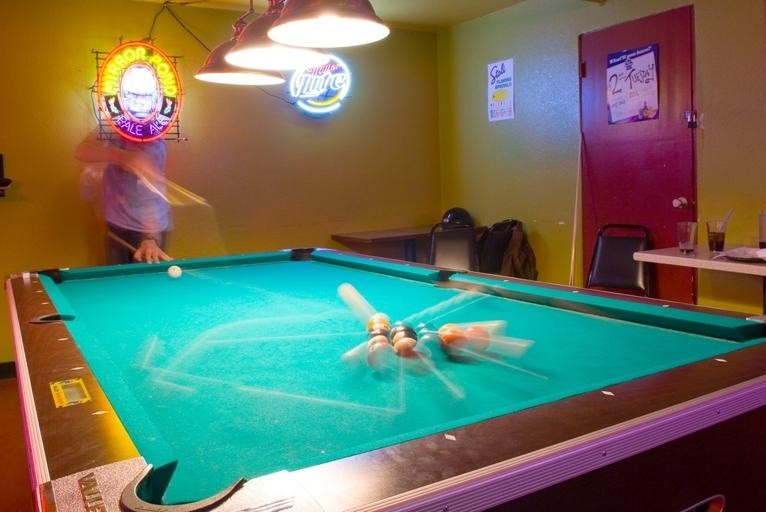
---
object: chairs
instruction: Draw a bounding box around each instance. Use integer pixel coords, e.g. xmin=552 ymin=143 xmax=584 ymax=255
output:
xmin=585 ymin=220 xmax=652 ymax=300
xmin=426 ymin=222 xmax=480 ymax=274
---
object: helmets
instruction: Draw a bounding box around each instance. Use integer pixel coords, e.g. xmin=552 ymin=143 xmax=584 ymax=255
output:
xmin=442 ymin=208 xmax=472 ymax=228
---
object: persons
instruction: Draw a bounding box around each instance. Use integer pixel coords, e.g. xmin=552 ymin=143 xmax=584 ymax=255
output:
xmin=73 ymin=124 xmax=173 ymax=265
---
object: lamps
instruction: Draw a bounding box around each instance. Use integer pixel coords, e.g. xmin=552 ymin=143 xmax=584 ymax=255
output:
xmin=192 ymin=1 xmax=388 ymax=87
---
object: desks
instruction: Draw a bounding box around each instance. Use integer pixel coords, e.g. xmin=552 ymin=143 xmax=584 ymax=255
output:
xmin=632 ymin=244 xmax=766 ymax=279
xmin=6 ymin=247 xmax=766 ymax=512
xmin=331 ymin=225 xmax=488 ymax=263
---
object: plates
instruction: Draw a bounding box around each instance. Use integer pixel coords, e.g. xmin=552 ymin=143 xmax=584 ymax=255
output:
xmin=722 ymin=252 xmax=756 ymax=261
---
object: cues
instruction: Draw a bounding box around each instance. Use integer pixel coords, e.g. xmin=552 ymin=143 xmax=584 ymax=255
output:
xmin=106 ymin=230 xmax=153 ymax=260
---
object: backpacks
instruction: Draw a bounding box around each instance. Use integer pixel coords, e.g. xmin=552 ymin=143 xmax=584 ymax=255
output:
xmin=478 ymin=219 xmax=538 ymax=280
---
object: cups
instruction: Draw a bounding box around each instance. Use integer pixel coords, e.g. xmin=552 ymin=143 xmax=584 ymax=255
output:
xmin=759 ymin=215 xmax=765 ymax=248
xmin=707 ymin=220 xmax=727 ymax=252
xmin=677 ymin=221 xmax=697 ymax=251
